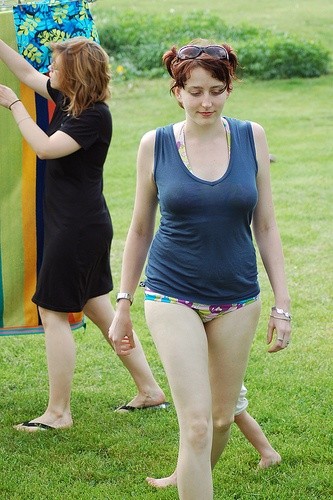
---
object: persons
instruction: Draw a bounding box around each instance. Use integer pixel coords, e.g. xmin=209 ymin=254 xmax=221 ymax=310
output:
xmin=147 ymin=382 xmax=282 ymax=489
xmin=0 ymin=38 xmax=170 ymax=435
xmin=108 ymin=40 xmax=294 ymax=500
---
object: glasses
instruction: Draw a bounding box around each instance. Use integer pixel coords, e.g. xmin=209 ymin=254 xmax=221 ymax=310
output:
xmin=176 ymin=45 xmax=231 ymax=62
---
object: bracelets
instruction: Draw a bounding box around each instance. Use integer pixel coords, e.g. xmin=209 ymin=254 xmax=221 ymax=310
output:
xmin=16 ymin=117 xmax=31 ymax=126
xmin=115 ymin=292 xmax=134 ymax=305
xmin=269 ymin=306 xmax=291 ymax=321
xmin=9 ymin=100 xmax=21 ymax=110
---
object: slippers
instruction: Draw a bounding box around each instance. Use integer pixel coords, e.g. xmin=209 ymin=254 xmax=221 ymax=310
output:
xmin=114 ymin=402 xmax=170 ymax=412
xmin=19 ymin=421 xmax=56 ymax=434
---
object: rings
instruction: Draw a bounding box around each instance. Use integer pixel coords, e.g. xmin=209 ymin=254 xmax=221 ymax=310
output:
xmin=277 ymin=338 xmax=291 ymax=343
